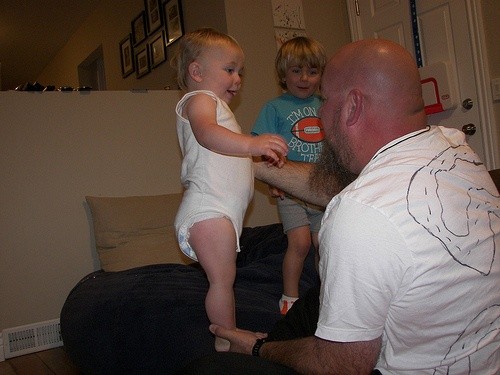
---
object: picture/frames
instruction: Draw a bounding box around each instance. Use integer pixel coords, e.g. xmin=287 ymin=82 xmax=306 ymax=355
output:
xmin=118 ymin=0 xmax=184 ymax=79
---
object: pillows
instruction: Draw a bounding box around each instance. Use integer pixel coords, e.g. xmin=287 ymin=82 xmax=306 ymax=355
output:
xmin=84 ymin=192 xmax=197 ymax=272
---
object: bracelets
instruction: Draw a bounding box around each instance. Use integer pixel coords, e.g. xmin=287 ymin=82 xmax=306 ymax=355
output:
xmin=251 ymin=337 xmax=270 ymax=357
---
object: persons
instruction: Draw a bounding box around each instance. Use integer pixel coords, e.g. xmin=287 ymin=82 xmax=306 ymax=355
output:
xmin=250 ymin=36 xmax=343 ymax=316
xmin=233 ymin=37 xmax=500 ymax=375
xmin=169 ymin=27 xmax=289 ymax=352
